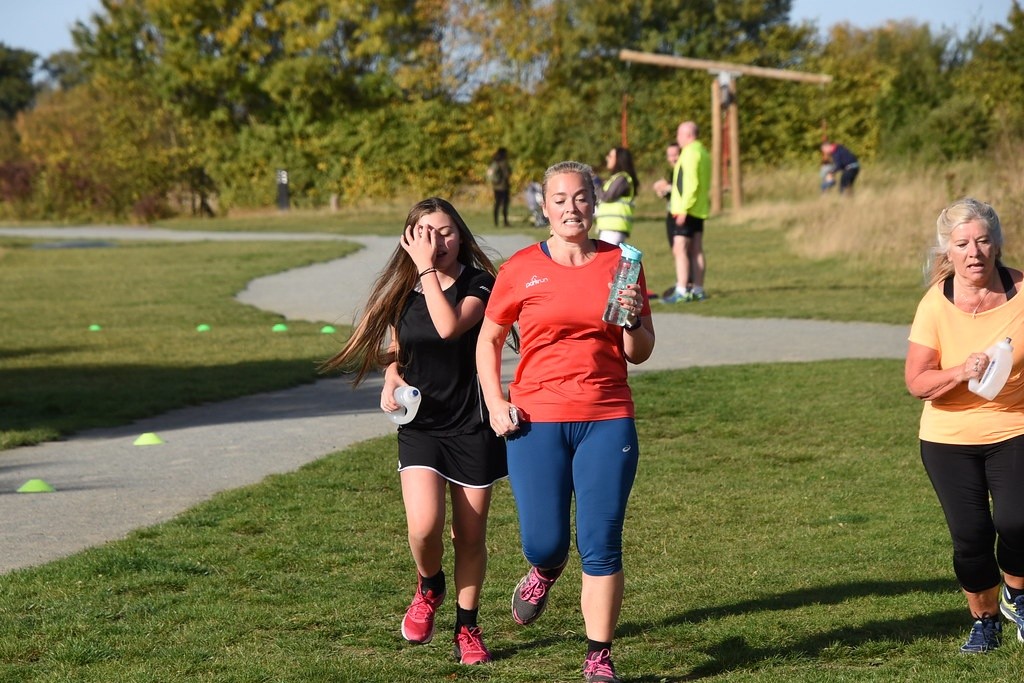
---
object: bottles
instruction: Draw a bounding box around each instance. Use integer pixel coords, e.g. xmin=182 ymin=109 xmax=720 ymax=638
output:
xmin=602 ymin=242 xmax=642 ymax=326
xmin=968 ymin=337 xmax=1013 ymax=401
xmin=385 ymin=386 xmax=421 ymax=424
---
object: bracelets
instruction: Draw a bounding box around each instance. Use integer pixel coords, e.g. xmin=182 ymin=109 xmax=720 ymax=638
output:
xmin=419 ymin=267 xmax=437 ymax=278
xmin=624 ymin=315 xmax=642 ymax=331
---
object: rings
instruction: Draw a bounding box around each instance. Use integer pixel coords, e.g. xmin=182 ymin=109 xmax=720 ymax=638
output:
xmin=976 ymin=358 xmax=979 ymax=365
xmin=974 ymin=366 xmax=978 ymax=371
xmin=634 ymin=299 xmax=638 ymax=307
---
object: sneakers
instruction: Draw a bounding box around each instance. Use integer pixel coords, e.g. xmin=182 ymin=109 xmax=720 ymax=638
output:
xmin=657 ymin=290 xmax=687 ymax=304
xmin=453 ymin=625 xmax=491 ymax=665
xmin=511 ymin=553 xmax=571 ymax=626
xmin=400 ymin=575 xmax=446 ymax=645
xmin=688 ymin=287 xmax=704 ymax=302
xmin=1000 ymin=585 xmax=1024 ymax=644
xmin=582 ymin=648 xmax=616 ymax=683
xmin=959 ymin=612 xmax=1002 ymax=656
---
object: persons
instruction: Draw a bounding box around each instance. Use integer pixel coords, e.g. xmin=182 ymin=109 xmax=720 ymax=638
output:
xmin=476 ymin=162 xmax=655 ymax=683
xmin=317 ymin=197 xmax=521 ymax=665
xmin=821 ymin=143 xmax=859 ymax=198
xmin=593 ymin=146 xmax=640 ymax=246
xmin=492 ymin=147 xmax=512 ymax=225
xmin=655 ymin=122 xmax=712 ymax=303
xmin=530 ymin=175 xmax=548 ymax=227
xmin=905 ymin=198 xmax=1024 ymax=652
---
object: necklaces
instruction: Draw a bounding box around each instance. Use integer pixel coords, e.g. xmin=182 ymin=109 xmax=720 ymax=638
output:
xmin=959 ymin=283 xmax=991 ymax=313
